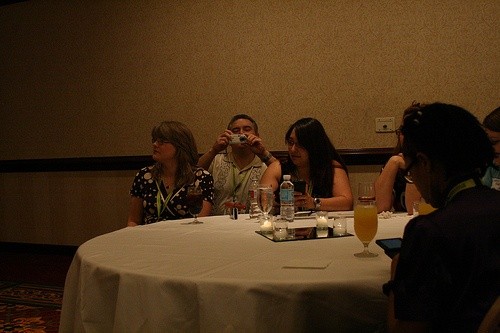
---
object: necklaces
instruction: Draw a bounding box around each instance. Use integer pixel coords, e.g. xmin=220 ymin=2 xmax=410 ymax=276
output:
xmin=164 ymin=181 xmax=173 ymax=189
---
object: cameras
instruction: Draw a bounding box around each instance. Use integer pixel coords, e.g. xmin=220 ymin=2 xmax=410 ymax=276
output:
xmin=229 ymin=134 xmax=247 ymax=144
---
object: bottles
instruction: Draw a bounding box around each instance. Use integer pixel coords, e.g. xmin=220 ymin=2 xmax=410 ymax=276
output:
xmin=248 ymin=177 xmax=263 ymax=220
xmin=280 ymin=175 xmax=295 ymax=220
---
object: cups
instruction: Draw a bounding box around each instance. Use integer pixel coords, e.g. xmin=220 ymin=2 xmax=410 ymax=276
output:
xmin=259 ymin=214 xmax=288 ymax=240
xmin=315 ymin=211 xmax=328 ymax=237
xmin=419 ymin=197 xmax=437 ymax=215
xmin=358 ymin=182 xmax=376 ymax=202
xmin=410 ymin=190 xmax=420 ymax=217
xmin=333 ymin=216 xmax=347 ymax=236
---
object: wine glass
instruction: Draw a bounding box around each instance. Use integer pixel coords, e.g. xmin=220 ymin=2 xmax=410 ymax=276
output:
xmin=256 ymin=184 xmax=274 ymax=222
xmin=187 ymin=187 xmax=204 ymax=224
xmin=354 ymin=200 xmax=380 ymax=257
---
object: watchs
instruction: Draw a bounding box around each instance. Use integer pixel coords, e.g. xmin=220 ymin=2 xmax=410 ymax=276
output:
xmin=313 ymin=197 xmax=321 ymax=210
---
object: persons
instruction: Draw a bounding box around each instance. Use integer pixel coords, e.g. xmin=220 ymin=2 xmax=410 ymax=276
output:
xmin=372 ymin=102 xmax=500 ymax=333
xmin=128 ymin=114 xmax=352 ymax=227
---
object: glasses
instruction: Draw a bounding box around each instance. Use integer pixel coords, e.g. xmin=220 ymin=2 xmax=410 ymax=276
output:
xmin=152 ymin=137 xmax=170 ymax=145
xmin=395 ymin=128 xmax=403 ymax=136
xmin=287 ymin=140 xmax=303 ymax=148
xmin=401 ymin=159 xmax=417 ymax=185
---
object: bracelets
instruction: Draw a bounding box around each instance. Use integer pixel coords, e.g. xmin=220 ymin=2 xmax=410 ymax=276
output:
xmin=261 ymin=153 xmax=272 ymax=163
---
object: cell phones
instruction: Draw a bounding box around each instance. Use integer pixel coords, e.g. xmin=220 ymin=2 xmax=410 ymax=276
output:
xmin=375 ymin=237 xmax=403 ymax=257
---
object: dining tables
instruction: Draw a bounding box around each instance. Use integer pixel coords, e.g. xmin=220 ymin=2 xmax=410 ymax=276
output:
xmin=58 ymin=211 xmax=418 ymax=332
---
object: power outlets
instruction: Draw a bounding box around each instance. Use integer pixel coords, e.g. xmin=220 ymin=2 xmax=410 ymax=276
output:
xmin=376 ymin=117 xmax=395 ymax=133
